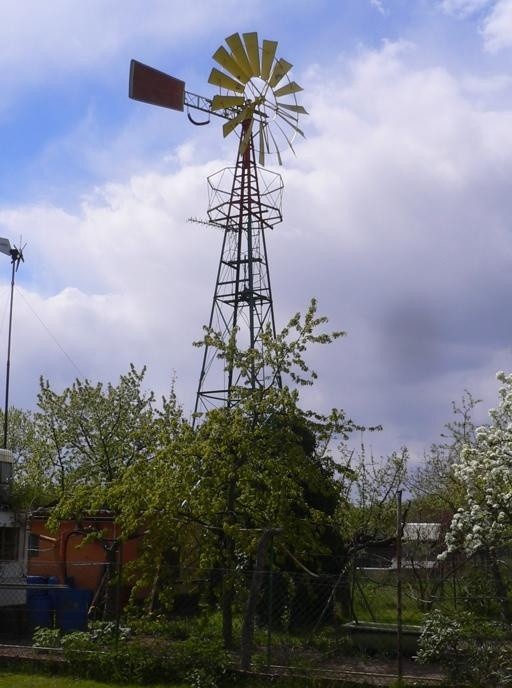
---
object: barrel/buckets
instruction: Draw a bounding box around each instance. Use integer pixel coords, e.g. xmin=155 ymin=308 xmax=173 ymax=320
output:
xmin=25 ymin=574 xmax=61 ymax=631
xmin=54 ymin=583 xmax=90 ymax=637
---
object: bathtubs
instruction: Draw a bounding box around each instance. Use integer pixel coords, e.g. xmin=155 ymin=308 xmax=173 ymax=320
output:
xmin=341 ymin=619 xmax=446 ymax=656
